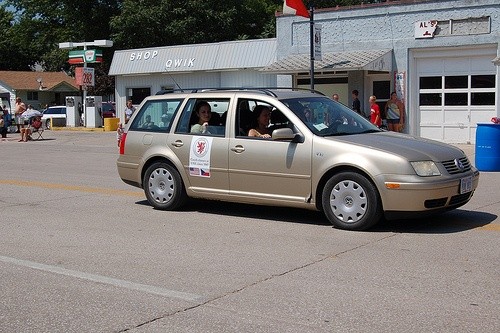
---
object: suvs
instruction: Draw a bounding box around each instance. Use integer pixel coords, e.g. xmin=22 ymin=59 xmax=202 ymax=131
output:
xmin=117 ymin=86 xmax=480 ymax=230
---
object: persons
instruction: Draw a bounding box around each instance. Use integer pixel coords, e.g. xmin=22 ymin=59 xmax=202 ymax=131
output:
xmin=385 ymin=91 xmax=407 ymax=130
xmin=0 ymin=88 xmax=383 ymax=134
xmin=247 ymin=105 xmax=271 ymax=139
xmin=189 ymin=102 xmax=212 ymax=135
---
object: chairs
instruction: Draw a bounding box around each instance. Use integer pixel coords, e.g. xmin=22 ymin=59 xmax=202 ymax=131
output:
xmin=188 ymin=112 xmax=228 ymax=135
xmin=270 ymin=109 xmax=291 ymax=137
xmin=240 ymin=108 xmax=255 ymax=137
xmin=28 ymin=122 xmax=44 ymax=140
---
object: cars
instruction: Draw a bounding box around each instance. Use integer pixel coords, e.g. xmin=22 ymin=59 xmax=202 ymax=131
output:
xmin=42 ymin=106 xmax=67 ymax=129
xmin=102 ymin=102 xmax=116 ymax=124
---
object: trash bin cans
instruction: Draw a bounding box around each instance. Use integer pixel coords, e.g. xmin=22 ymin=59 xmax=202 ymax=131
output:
xmin=474 ymin=123 xmax=500 ymax=171
